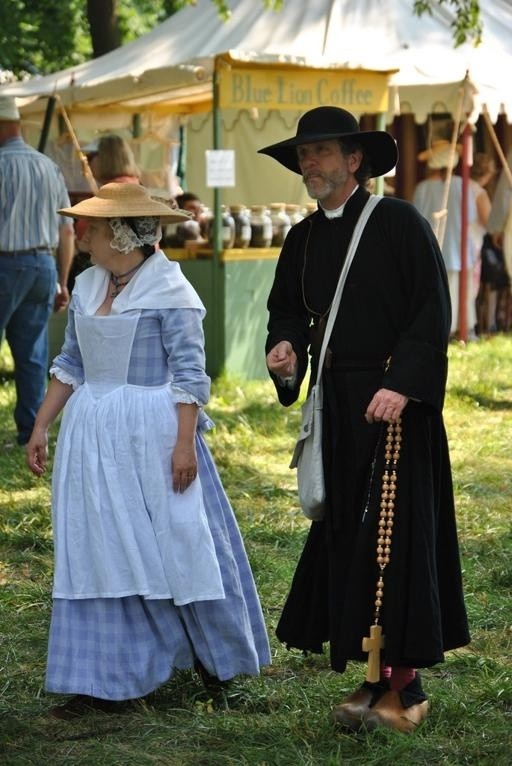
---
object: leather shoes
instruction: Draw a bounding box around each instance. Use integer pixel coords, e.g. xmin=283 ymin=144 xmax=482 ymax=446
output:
xmin=333 ymin=674 xmax=428 ymax=731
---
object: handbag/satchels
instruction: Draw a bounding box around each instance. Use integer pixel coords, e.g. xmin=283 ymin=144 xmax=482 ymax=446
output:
xmin=289 ymin=385 xmax=327 ymax=520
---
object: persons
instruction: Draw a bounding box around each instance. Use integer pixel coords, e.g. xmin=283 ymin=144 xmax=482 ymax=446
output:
xmin=487 ymin=153 xmax=512 ymax=279
xmin=252 ymin=106 xmax=470 ymax=740
xmin=25 ymin=184 xmax=272 ymax=718
xmin=66 ymin=132 xmax=160 ymax=299
xmin=415 ymin=140 xmax=474 ymax=341
xmin=0 ymin=96 xmax=75 ymax=448
xmin=175 ymin=192 xmax=201 ymax=220
xmin=469 ymin=152 xmax=495 ymax=341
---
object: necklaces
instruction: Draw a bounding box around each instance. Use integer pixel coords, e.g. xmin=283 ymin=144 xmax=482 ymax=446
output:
xmin=360 ymin=413 xmax=402 ymax=683
xmin=301 ymin=217 xmax=343 ymax=325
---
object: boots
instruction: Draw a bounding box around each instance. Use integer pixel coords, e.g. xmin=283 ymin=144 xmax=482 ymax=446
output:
xmin=48 ymin=694 xmax=119 ymax=720
xmin=203 ymin=676 xmax=240 ymax=703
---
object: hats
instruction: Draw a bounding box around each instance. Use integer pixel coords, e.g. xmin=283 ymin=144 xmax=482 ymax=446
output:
xmin=257 ymin=106 xmax=399 ymax=178
xmin=417 ymin=140 xmax=463 ymax=169
xmin=0 ymin=96 xmax=20 ymax=120
xmin=56 ymin=182 xmax=192 ymax=225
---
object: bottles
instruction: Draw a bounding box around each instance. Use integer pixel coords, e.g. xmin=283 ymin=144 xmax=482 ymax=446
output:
xmin=177 ymin=202 xmax=318 ymax=249
